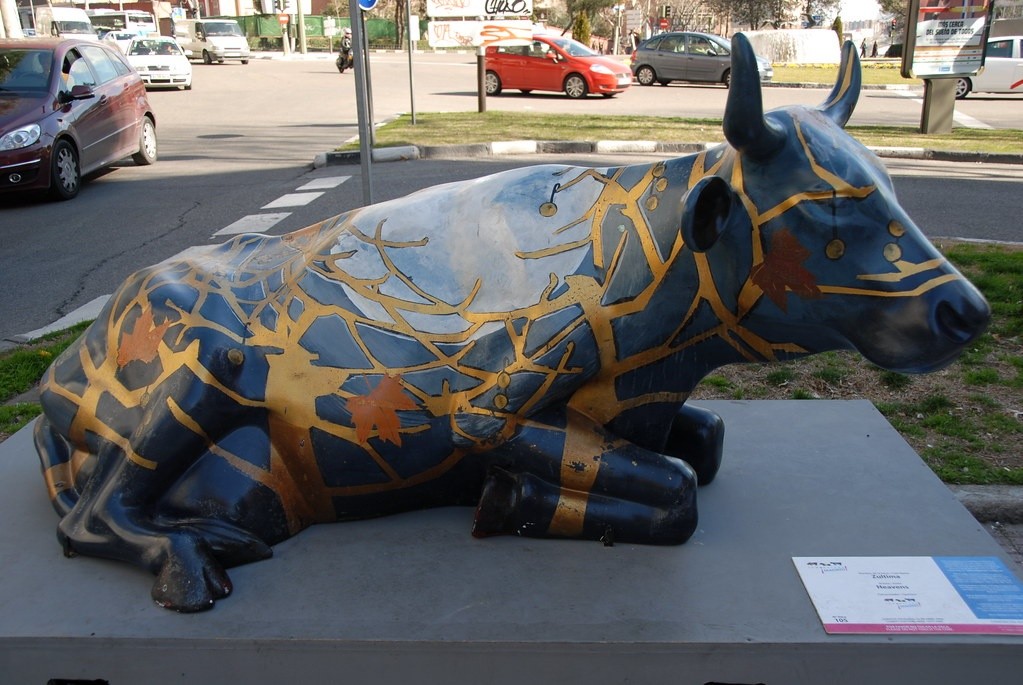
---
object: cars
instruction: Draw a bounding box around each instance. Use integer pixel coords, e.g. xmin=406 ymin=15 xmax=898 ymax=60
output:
xmin=481 ymin=36 xmax=633 ymax=99
xmin=955 ymin=35 xmax=1023 ymax=99
xmin=0 ymin=35 xmax=161 ymax=203
xmin=628 ymin=33 xmax=775 ymax=87
xmin=102 ymin=30 xmax=193 ymax=90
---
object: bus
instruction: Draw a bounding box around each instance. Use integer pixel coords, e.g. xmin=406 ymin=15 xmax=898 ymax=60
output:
xmin=87 ymin=8 xmax=158 ymax=32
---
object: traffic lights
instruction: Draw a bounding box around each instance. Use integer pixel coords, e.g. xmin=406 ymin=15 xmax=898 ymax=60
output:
xmin=892 ymin=20 xmax=896 ymax=30
xmin=666 ymin=6 xmax=671 ymax=17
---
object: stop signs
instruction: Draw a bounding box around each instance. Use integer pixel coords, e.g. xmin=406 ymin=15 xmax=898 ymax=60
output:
xmin=278 ymin=14 xmax=289 ymax=24
xmin=659 ymin=19 xmax=669 ymax=28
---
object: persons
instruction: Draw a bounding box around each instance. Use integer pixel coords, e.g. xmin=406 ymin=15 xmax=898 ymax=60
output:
xmin=860 ymin=38 xmax=867 ymax=58
xmin=693 ymin=39 xmax=711 ymax=54
xmin=161 ymin=42 xmax=178 ymax=56
xmin=342 ymin=28 xmax=353 ymax=59
xmin=37 ymin=50 xmax=74 ymax=93
xmin=870 ymin=40 xmax=878 ymax=57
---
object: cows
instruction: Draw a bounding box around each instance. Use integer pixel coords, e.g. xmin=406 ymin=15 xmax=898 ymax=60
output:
xmin=32 ymin=32 xmax=992 ymax=616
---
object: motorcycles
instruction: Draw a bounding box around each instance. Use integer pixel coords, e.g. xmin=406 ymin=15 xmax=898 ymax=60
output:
xmin=334 ymin=49 xmax=355 ymax=74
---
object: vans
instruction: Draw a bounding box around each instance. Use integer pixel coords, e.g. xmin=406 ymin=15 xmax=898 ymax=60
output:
xmin=36 ymin=6 xmax=99 ymax=40
xmin=173 ymin=18 xmax=249 ymax=65
